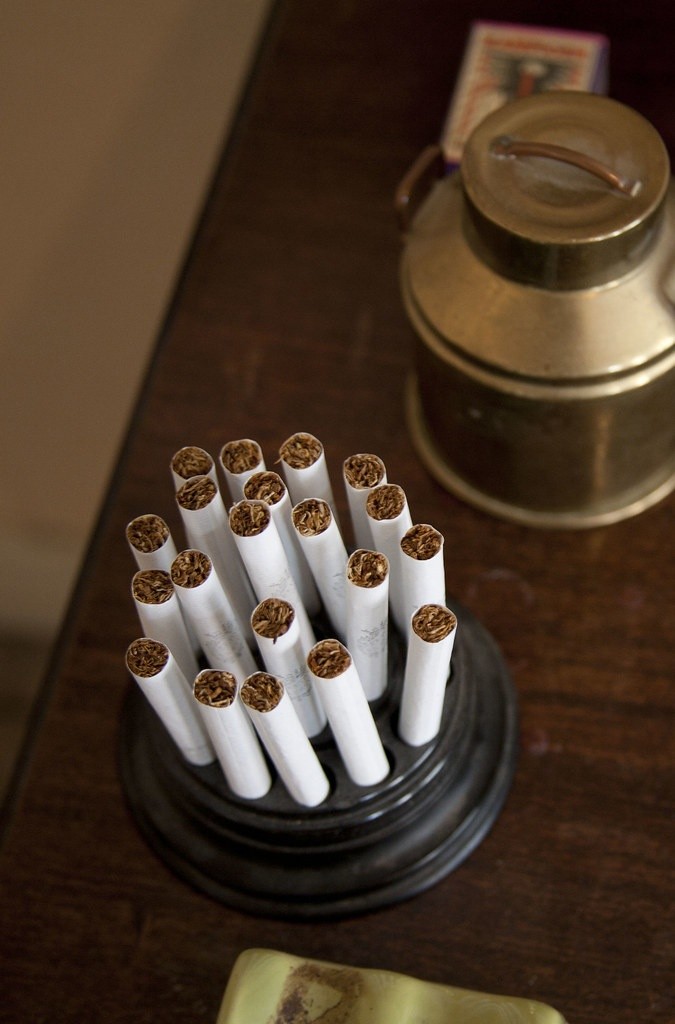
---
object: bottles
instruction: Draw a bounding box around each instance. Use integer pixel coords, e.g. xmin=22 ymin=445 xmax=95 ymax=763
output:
xmin=390 ymin=89 xmax=675 ymax=531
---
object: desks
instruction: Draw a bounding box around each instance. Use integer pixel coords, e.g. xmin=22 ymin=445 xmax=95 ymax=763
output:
xmin=3 ymin=0 xmax=675 ymax=1023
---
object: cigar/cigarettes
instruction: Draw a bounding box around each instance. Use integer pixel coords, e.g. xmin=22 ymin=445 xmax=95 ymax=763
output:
xmin=127 ymin=431 xmax=459 ymax=807
